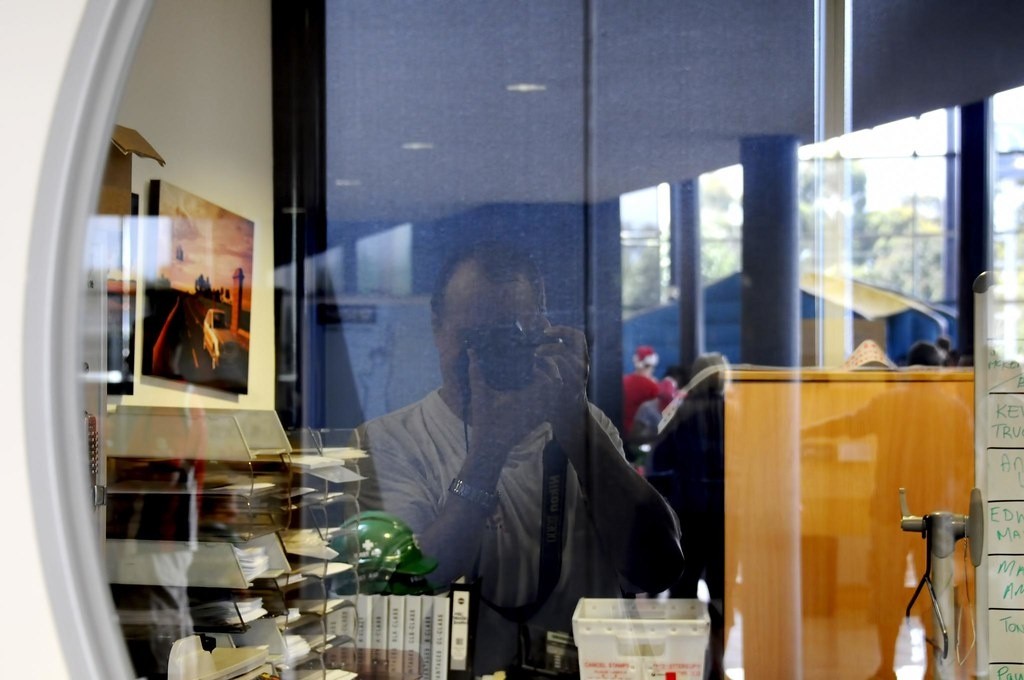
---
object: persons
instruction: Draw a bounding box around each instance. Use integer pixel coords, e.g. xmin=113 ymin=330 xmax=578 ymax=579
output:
xmin=644 ymin=352 xmax=743 ymax=680
xmin=803 ymin=356 xmax=973 ymax=679
xmin=187 ymin=480 xmax=256 ymax=646
xmin=346 ymin=242 xmax=692 ymax=680
xmin=621 ymin=329 xmax=975 ymax=471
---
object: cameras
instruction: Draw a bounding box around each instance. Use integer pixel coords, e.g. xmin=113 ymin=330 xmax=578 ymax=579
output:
xmin=449 ymin=319 xmax=566 ymax=414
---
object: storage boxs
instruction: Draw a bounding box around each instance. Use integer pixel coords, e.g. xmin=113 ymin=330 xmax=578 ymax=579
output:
xmin=570 ymin=596 xmax=711 ymax=680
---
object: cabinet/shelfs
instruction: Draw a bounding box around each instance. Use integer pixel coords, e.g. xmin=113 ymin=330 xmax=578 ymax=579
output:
xmin=99 ymin=402 xmax=364 ymax=680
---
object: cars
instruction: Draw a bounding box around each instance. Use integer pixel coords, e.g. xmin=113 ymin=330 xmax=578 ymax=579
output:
xmin=202 ymin=309 xmax=240 ymax=371
